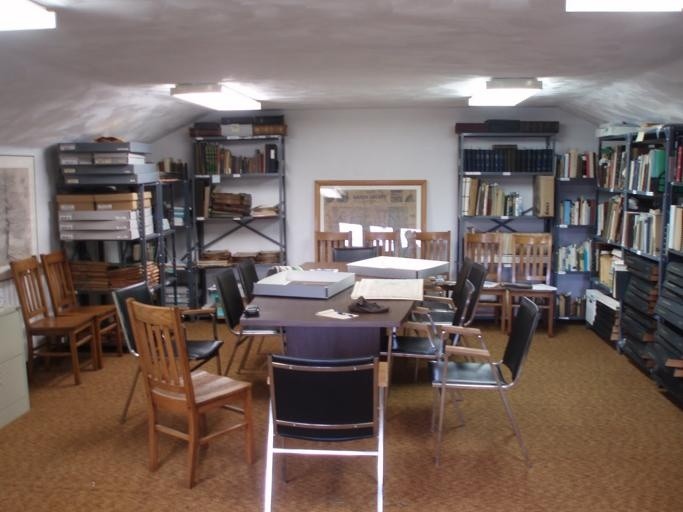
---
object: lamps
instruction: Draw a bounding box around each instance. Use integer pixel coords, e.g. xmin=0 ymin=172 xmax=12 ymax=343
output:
xmin=468 ymin=77 xmax=543 ymax=108
xmin=169 ymin=82 xmax=261 ymax=112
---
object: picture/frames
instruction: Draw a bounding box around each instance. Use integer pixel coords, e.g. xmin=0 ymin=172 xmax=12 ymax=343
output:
xmin=1 ymin=154 xmax=36 ymax=284
xmin=314 ymin=179 xmax=426 ymax=259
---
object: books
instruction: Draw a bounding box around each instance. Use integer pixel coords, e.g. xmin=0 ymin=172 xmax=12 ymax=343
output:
xmin=622 ymin=207 xmax=661 ymax=258
xmin=202 ymin=185 xmax=279 ymax=219
xmin=628 ymin=149 xmax=666 ymax=193
xmin=667 ymin=204 xmax=683 ymax=251
xmin=462 ymin=177 xmax=523 ymax=217
xmin=463 ymin=144 xmax=554 ymax=173
xmin=194 ymin=141 xmax=279 ymax=174
xmin=654 ymin=260 xmax=683 ymax=378
xmin=602 ymin=145 xmax=626 ymax=189
xmin=597 ymin=194 xmax=622 ymax=248
xmin=557 ymin=147 xmax=599 ymax=178
xmin=599 ymin=249 xmax=628 ymax=299
xmin=559 ymin=239 xmax=594 ymax=271
xmin=592 ymin=301 xmax=619 ymax=342
xmin=621 ymin=253 xmax=659 ymax=368
xmin=555 ymin=292 xmax=587 ymax=317
xmin=199 ymin=251 xmax=280 ymax=266
xmin=557 ymin=195 xmax=596 ymax=226
xmin=669 ymin=141 xmax=683 ymax=182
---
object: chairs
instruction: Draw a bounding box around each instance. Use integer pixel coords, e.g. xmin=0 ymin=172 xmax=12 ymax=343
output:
xmin=313 ymin=231 xmax=352 ymax=263
xmin=112 ymin=281 xmax=223 ymax=424
xmin=392 ymin=279 xmax=474 ymax=405
xmin=462 ymin=232 xmax=506 ymax=334
xmin=217 ymin=269 xmax=285 ymax=378
xmin=40 ymin=251 xmax=121 ymax=371
xmin=411 ymin=255 xmax=475 ymax=311
xmin=264 ymin=353 xmax=385 ymax=512
xmin=406 ymin=262 xmax=488 ymax=383
xmin=430 ymin=296 xmax=539 ymax=465
xmin=412 ymin=231 xmax=450 ymax=305
xmin=10 ymin=256 xmax=98 ymax=386
xmin=507 ymin=233 xmax=558 ymax=337
xmin=126 ymin=297 xmax=255 ymax=488
xmin=364 ymin=229 xmax=400 ymax=259
xmin=236 ymin=259 xmax=261 ymax=306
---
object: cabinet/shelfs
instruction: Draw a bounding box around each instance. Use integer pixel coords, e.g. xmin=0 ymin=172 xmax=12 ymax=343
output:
xmin=556 ymin=173 xmax=593 ymax=320
xmin=593 ymin=126 xmax=681 ymax=381
xmin=459 ymin=131 xmax=554 ymax=321
xmin=56 ymin=177 xmax=189 ymax=345
xmin=192 ymin=136 xmax=289 ymax=323
